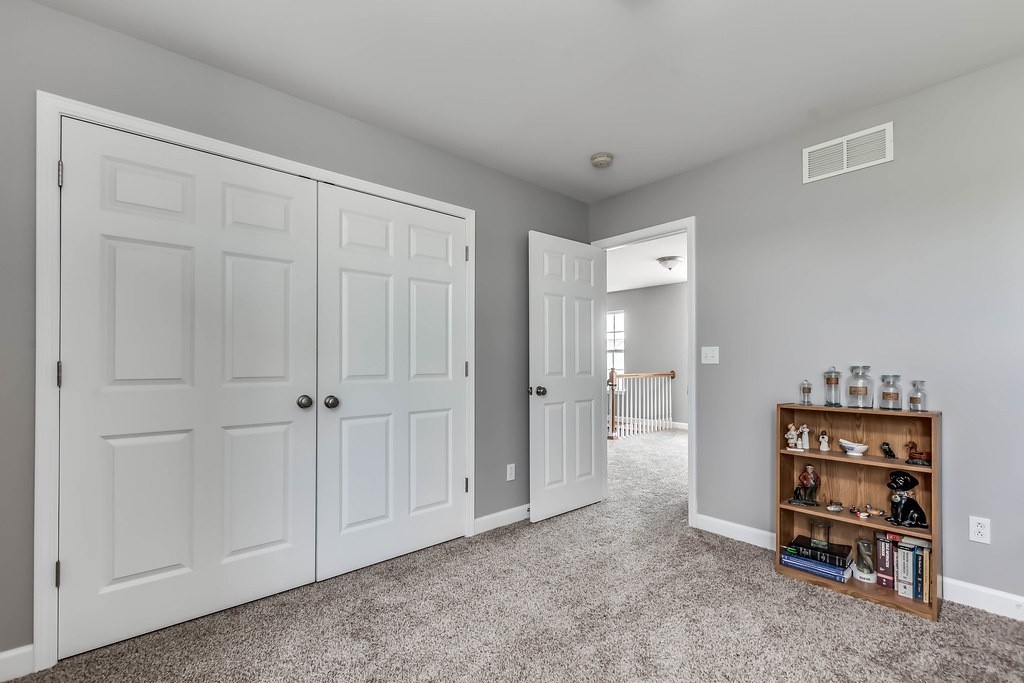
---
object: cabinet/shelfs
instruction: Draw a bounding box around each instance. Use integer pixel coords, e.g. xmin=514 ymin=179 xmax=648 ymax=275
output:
xmin=774 ymin=403 xmax=944 ymax=622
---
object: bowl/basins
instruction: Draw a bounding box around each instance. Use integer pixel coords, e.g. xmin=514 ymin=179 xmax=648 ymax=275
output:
xmin=839 ymin=443 xmax=869 ymax=455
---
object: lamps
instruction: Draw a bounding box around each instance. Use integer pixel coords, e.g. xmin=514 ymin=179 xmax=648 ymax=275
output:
xmin=656 ymin=256 xmax=683 ymax=271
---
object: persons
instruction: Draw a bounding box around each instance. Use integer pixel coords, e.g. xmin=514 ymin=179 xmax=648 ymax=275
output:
xmin=819 ymin=431 xmax=831 ymax=451
xmin=785 ymin=423 xmax=799 ymax=448
xmin=800 ymin=424 xmax=810 ymax=449
xmin=799 ymin=464 xmax=821 ymax=502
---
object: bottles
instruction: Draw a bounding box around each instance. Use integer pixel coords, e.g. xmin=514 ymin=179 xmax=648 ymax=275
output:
xmin=879 ymin=375 xmax=903 ymax=409
xmin=823 ymin=366 xmax=842 ymax=408
xmin=846 ymin=365 xmax=875 ymax=409
xmin=799 ymin=380 xmax=813 ymax=407
xmin=910 ymin=380 xmax=928 ymax=412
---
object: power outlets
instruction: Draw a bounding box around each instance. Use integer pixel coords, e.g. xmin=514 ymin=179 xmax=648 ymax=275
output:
xmin=967 ymin=515 xmax=991 ymax=545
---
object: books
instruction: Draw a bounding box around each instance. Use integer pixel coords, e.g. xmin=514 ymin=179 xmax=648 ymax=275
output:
xmin=780 ymin=535 xmax=854 ymax=583
xmin=876 ymin=533 xmax=933 ymax=604
